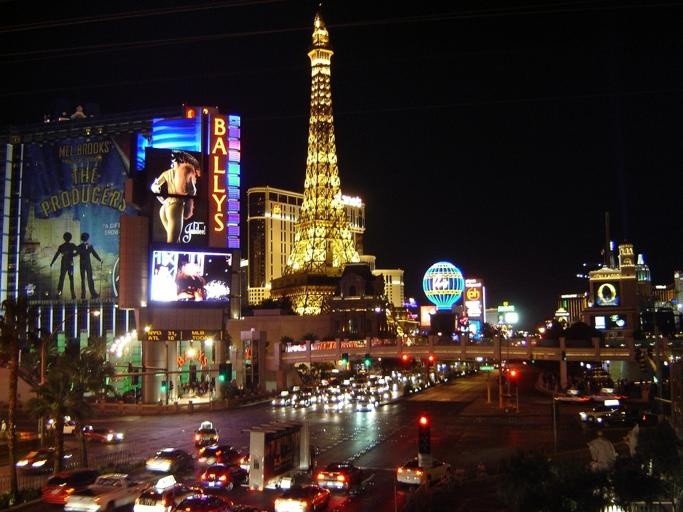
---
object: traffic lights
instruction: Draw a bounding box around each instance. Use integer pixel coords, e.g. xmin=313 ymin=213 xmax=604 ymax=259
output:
xmin=218 ymin=363 xmax=226 ymax=383
xmin=401 ymin=353 xmax=435 ymax=366
xmin=418 ymin=415 xmax=431 ymax=455
xmin=338 ymin=352 xmax=371 ymax=367
xmin=510 ymin=369 xmax=522 ymax=381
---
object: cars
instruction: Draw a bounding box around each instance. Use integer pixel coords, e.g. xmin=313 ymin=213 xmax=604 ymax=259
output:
xmin=578 ymin=404 xmax=652 ymax=427
xmin=265 ymin=462 xmax=376 ymax=512
xmin=272 ymin=372 xmax=422 ymax=411
xmin=16 ymin=416 xmax=260 ymax=512
xmin=397 ymin=455 xmax=451 ymax=488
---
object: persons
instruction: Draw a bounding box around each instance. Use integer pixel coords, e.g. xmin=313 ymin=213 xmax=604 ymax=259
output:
xmin=49 ymin=231 xmax=104 ymax=300
xmin=175 ymin=259 xmax=205 ymax=301
xmin=182 ymin=381 xmax=214 ymax=393
xmin=150 ymin=151 xmax=200 ymax=243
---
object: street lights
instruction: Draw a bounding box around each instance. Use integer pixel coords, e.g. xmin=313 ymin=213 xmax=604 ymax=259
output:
xmin=40 ymin=307 xmax=102 ymax=447
